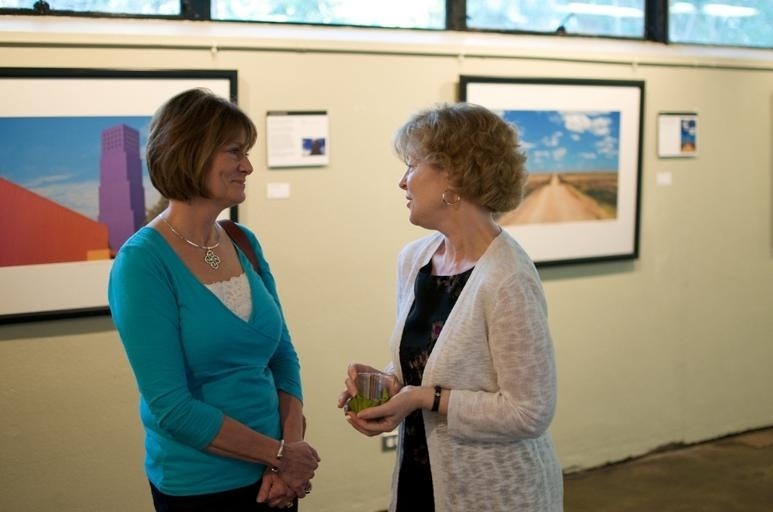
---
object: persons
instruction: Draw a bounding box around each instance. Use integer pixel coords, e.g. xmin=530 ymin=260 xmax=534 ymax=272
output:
xmin=106 ymin=85 xmax=321 ymax=511
xmin=335 ymin=101 xmax=567 ymax=512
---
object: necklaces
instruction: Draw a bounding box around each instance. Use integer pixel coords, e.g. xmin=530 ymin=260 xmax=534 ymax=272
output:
xmin=158 ymin=211 xmax=224 ymax=271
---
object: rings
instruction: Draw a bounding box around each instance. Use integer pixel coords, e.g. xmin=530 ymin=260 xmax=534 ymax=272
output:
xmin=304 ymin=482 xmax=311 ymax=494
xmin=286 ymin=501 xmax=294 ymax=508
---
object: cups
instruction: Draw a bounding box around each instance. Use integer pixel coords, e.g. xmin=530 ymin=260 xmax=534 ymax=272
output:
xmin=348 ymin=372 xmax=396 ymax=416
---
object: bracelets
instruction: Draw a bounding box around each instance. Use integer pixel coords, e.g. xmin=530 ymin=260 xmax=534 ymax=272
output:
xmin=430 ymin=385 xmax=440 ymax=413
xmin=271 ymin=438 xmax=285 ymax=475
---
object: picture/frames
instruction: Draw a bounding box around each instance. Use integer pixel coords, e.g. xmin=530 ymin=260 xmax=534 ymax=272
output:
xmin=459 ymin=75 xmax=644 ymax=267
xmin=1 ymin=67 xmax=238 ymax=324
